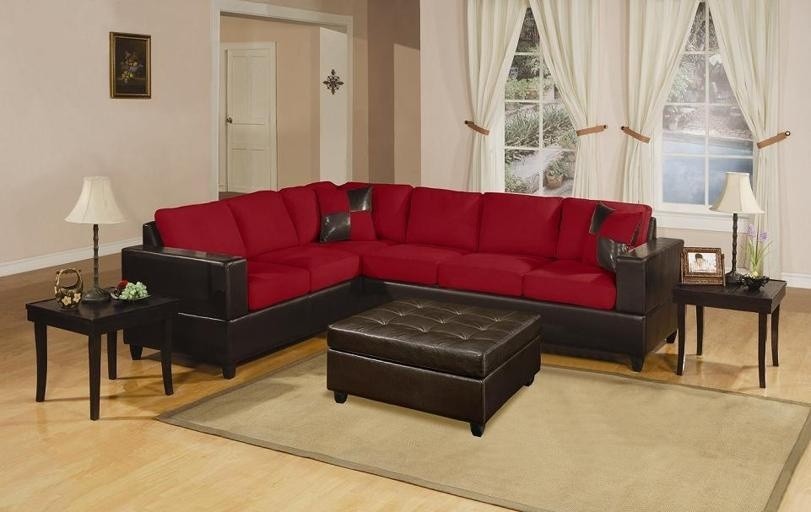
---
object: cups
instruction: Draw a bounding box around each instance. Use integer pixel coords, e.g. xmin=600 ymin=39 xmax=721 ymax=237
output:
xmin=739 ymin=274 xmax=770 ymax=291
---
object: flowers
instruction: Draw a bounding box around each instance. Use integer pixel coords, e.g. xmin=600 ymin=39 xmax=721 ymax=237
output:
xmin=741 ymin=223 xmax=774 ymax=277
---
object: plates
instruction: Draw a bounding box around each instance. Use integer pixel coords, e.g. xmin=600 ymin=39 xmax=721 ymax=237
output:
xmin=110 ymin=288 xmax=151 ymax=302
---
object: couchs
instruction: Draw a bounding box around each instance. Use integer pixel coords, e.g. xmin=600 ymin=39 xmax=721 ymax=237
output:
xmin=120 ymin=179 xmax=686 ymax=382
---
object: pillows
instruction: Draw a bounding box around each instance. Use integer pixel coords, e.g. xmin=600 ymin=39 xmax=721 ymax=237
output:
xmin=581 ymin=201 xmax=643 ymax=275
xmin=314 ymin=185 xmax=377 ymax=247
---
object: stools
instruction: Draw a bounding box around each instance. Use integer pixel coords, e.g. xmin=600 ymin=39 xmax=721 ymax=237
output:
xmin=325 ymin=295 xmax=542 ymax=437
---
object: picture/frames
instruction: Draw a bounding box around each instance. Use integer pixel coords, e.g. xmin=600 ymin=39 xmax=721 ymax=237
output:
xmin=108 ymin=30 xmax=153 ymax=101
xmin=679 ymin=245 xmax=725 ymax=288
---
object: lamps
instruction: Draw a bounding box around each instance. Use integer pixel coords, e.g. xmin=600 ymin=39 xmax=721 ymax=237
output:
xmin=63 ymin=172 xmax=129 ymax=305
xmin=708 ymin=171 xmax=768 ymax=288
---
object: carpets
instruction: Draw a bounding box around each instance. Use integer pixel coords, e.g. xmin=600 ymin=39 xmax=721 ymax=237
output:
xmin=153 ymin=347 xmax=811 ymax=510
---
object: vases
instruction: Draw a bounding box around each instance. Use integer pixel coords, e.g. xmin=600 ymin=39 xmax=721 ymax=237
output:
xmin=741 ymin=273 xmax=770 ymax=290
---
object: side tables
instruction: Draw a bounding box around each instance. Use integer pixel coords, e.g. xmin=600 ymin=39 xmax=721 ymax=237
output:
xmin=671 ymin=274 xmax=787 ymax=387
xmin=24 ymin=285 xmax=179 ymax=423
